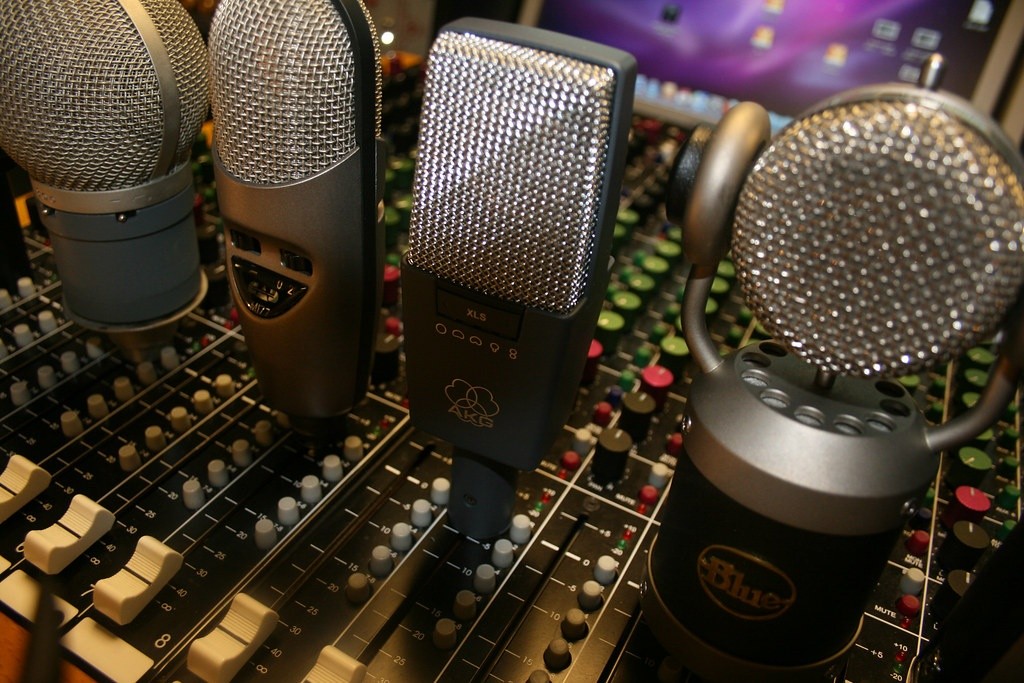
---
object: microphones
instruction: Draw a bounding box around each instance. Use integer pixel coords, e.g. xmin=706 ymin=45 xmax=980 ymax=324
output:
xmin=207 ymin=1 xmax=388 ymax=420
xmin=647 ymin=82 xmax=1024 ymax=672
xmin=0 ymin=0 xmax=206 ymax=367
xmin=398 ymin=16 xmax=638 ymax=540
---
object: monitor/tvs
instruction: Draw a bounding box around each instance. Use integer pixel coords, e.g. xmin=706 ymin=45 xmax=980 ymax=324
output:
xmin=515 ymin=0 xmax=1023 ymax=135
